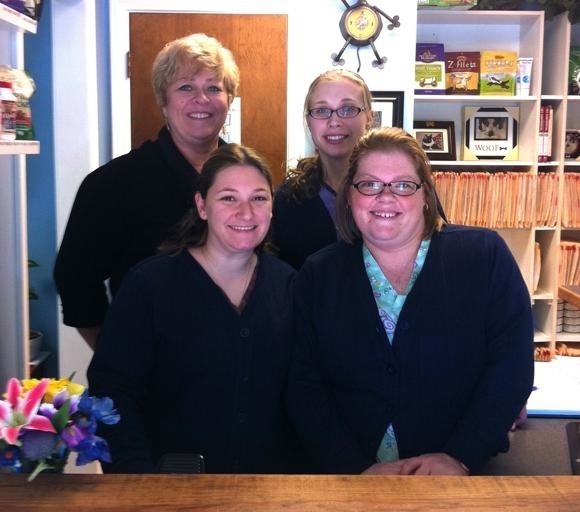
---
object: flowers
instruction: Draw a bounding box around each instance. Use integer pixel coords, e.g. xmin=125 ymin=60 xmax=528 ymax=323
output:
xmin=0 ymin=370 xmax=122 ymax=484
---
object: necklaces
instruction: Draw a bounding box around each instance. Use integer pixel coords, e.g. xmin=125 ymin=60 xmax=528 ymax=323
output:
xmin=380 ymin=241 xmax=420 ymax=287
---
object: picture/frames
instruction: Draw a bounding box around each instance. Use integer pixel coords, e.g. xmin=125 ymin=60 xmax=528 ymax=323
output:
xmin=461 ymin=105 xmax=521 ymax=161
xmin=412 ymin=119 xmax=458 ymax=161
xmin=367 ymin=90 xmax=404 ymax=131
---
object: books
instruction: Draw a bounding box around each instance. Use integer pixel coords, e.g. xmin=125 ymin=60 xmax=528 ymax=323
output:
xmin=417 ymin=40 xmax=553 ymax=165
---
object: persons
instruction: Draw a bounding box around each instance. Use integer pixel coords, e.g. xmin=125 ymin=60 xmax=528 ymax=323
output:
xmin=51 ymin=30 xmax=243 ymax=354
xmin=297 ymin=126 xmax=537 ymax=475
xmin=83 ymin=140 xmax=422 ymax=477
xmin=270 ymin=67 xmax=529 ymax=432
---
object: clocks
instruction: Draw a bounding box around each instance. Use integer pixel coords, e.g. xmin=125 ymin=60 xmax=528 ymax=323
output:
xmin=331 ymin=0 xmax=400 ymax=73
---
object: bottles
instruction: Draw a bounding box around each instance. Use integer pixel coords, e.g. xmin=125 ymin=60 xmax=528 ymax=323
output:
xmin=0 ymin=81 xmax=19 ymax=141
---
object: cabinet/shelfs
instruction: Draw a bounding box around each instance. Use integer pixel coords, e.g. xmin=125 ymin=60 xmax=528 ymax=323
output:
xmin=0 ymin=1 xmax=41 ymax=393
xmin=412 ymin=8 xmax=580 ymax=356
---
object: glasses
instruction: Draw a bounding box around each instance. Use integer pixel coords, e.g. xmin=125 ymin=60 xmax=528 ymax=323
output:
xmin=348 ymin=178 xmax=424 ymax=197
xmin=306 ymin=106 xmax=366 ymax=121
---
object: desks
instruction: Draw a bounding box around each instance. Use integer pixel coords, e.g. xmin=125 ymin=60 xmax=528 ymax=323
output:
xmin=0 ymin=466 xmax=579 ymax=511
